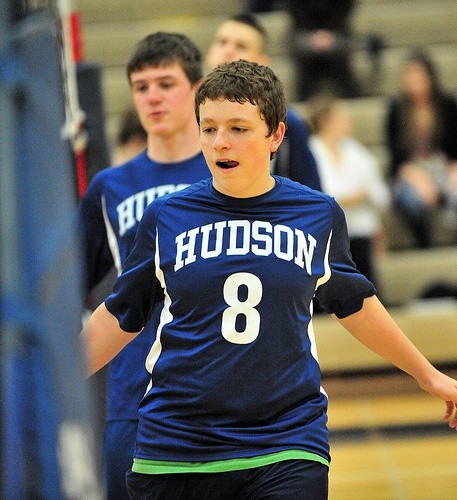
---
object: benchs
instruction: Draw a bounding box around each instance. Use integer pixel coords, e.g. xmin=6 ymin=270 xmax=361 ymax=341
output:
xmin=76 ymin=0 xmax=457 ymax=383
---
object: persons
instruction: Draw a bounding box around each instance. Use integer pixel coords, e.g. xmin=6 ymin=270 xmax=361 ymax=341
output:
xmin=74 ymin=31 xmax=211 ymax=500
xmin=111 ymin=0 xmax=457 ymax=298
xmin=78 ymin=57 xmax=456 ymax=499
